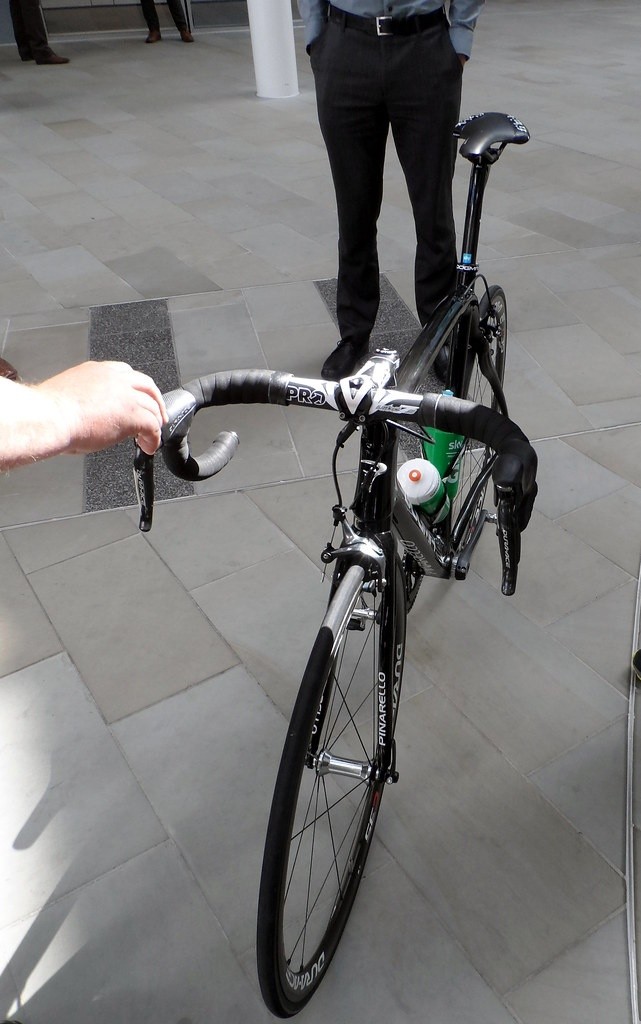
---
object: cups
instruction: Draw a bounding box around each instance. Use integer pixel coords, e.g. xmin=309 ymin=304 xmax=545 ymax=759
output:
xmin=396 ymin=458 xmax=451 ymax=525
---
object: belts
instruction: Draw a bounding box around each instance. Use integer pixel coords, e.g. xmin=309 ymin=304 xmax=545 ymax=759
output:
xmin=326 ymin=4 xmax=446 ymax=36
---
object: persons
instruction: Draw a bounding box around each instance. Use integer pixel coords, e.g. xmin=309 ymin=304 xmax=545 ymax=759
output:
xmin=9 ymin=0 xmax=69 ymax=64
xmin=0 ymin=359 xmax=168 ymax=473
xmin=141 ymin=0 xmax=194 ymax=43
xmin=297 ymin=0 xmax=480 ymax=386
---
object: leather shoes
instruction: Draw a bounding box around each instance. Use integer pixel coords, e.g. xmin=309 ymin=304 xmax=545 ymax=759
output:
xmin=36 ymin=52 xmax=70 ymax=64
xmin=19 ymin=48 xmax=37 ymax=61
xmin=180 ymin=31 xmax=194 ymax=42
xmin=145 ymin=31 xmax=162 ymax=43
xmin=427 ymin=321 xmax=455 ymax=383
xmin=319 ymin=334 xmax=371 ymax=379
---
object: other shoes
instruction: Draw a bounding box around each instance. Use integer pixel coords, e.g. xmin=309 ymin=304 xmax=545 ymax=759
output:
xmin=0 ymin=357 xmax=19 ymax=380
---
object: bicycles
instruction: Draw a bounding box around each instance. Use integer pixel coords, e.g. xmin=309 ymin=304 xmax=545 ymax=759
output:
xmin=133 ymin=112 xmax=538 ymax=1019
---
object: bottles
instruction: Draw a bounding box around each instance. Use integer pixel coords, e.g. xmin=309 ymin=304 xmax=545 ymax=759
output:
xmin=421 ymin=390 xmax=465 ymax=499
